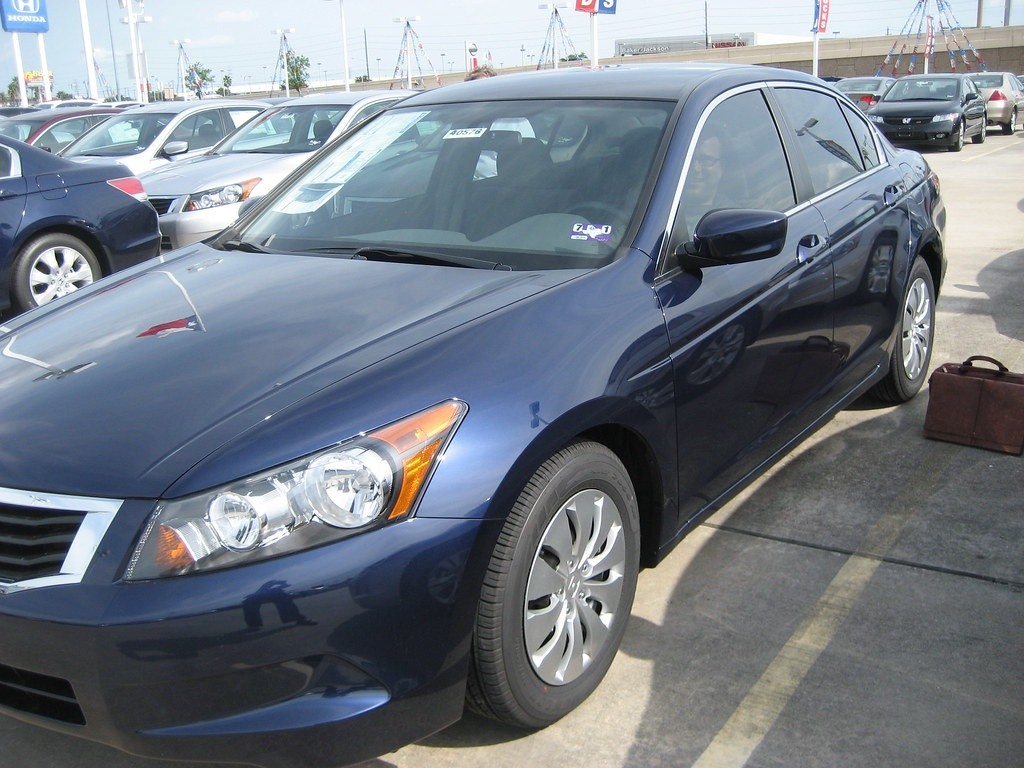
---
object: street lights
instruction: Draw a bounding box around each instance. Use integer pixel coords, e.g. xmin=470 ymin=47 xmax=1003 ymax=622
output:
xmin=537 ymin=2 xmax=569 ymax=69
xmin=120 ymin=13 xmax=153 ymax=52
xmin=394 ymin=13 xmax=420 ymax=90
xmin=271 ymin=27 xmax=296 ymax=95
xmin=171 ymin=37 xmax=190 ymax=101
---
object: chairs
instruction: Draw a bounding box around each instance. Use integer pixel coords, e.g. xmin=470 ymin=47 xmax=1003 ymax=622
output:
xmin=313 ymin=119 xmax=334 ymax=146
xmin=598 ymin=125 xmax=669 ymax=232
xmin=198 ymin=123 xmax=221 ymax=148
xmin=154 ymin=125 xmax=175 ymax=150
xmin=475 ymin=135 xmax=554 ymax=240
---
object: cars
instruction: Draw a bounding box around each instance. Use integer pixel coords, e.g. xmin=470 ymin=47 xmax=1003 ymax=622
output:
xmin=961 ymin=72 xmax=1024 ymax=136
xmin=50 ymin=98 xmax=275 ymax=177
xmin=1 ymin=133 xmax=165 ymax=324
xmin=136 ymin=92 xmax=426 ymax=253
xmin=832 ymin=76 xmax=898 ymax=124
xmin=3 ymin=60 xmax=948 ymax=768
xmin=864 ymin=72 xmax=988 ymax=152
xmin=1 ymin=97 xmax=141 ymax=160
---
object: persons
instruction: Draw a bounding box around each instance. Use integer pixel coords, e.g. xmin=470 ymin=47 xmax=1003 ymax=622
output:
xmin=679 ymin=125 xmax=744 ymax=245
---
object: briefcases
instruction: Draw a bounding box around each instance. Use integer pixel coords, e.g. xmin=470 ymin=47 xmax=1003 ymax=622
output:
xmin=922 ymin=355 xmax=1024 ymax=456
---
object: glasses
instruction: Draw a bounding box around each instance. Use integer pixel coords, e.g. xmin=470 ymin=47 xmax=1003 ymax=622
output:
xmin=690 ymin=156 xmax=723 ymax=168
